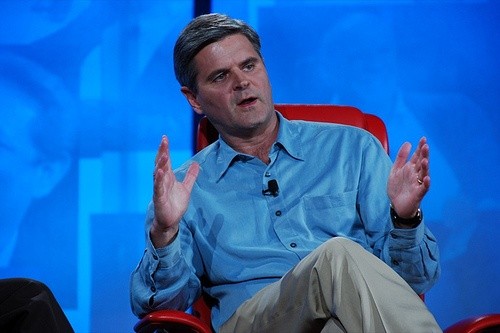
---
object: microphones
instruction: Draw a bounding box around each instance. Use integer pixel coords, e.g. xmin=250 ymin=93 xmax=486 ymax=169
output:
xmin=262 ymin=179 xmax=278 ymax=196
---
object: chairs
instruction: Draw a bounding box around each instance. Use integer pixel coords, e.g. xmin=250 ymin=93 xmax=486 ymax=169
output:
xmin=0 ymin=277 xmax=76 ymax=333
xmin=133 ymin=103 xmax=500 ymax=333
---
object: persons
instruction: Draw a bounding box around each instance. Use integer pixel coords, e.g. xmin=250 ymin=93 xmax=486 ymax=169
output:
xmin=130 ymin=13 xmax=445 ymax=333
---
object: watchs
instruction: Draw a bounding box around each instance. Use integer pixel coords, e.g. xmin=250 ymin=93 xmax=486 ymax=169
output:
xmin=389 ymin=204 xmax=421 ymax=225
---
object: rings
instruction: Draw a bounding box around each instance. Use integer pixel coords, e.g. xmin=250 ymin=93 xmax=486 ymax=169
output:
xmin=417 ymin=175 xmax=423 ymax=184
